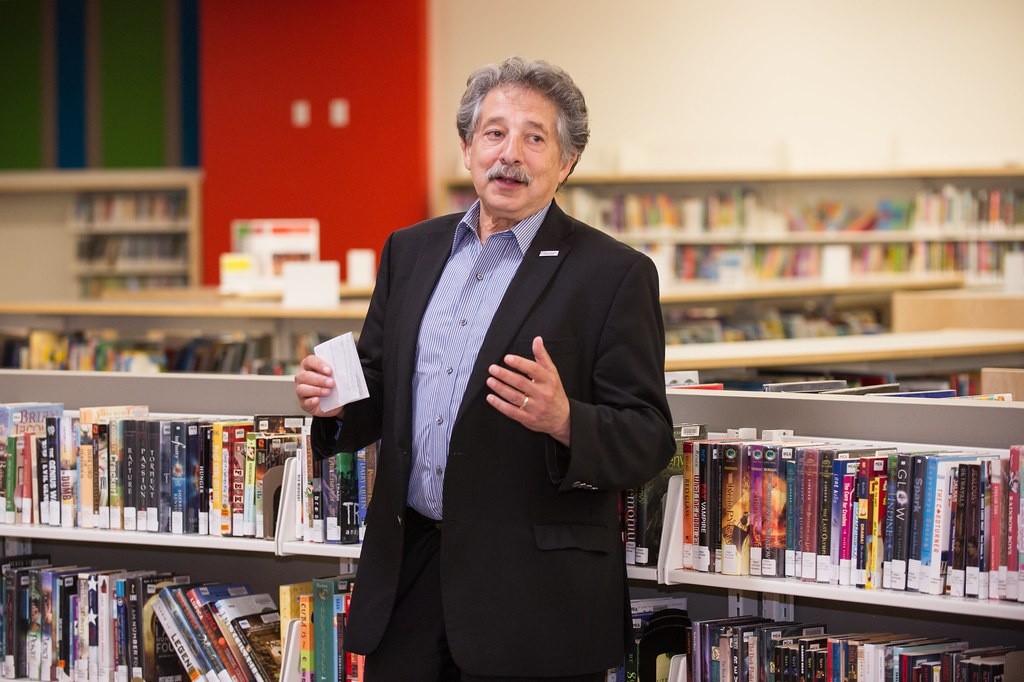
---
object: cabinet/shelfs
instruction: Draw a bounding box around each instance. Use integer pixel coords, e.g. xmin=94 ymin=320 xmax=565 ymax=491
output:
xmin=444 ymin=165 xmax=1024 ymax=280
xmin=0 ymin=368 xmax=1024 ymax=682
xmin=0 ymin=171 xmax=203 ymax=297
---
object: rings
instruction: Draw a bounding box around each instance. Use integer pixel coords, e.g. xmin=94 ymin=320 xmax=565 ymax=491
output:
xmin=520 ymin=396 xmax=528 ymax=409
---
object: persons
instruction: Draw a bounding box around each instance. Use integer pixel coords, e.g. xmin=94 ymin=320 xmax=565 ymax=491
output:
xmin=294 ymin=56 xmax=677 ymax=681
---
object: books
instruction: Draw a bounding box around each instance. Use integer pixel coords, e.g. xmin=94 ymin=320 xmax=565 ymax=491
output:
xmin=641 ymin=242 xmax=1024 ymax=284
xmin=0 ymin=554 xmax=367 ymax=682
xmin=75 ymin=233 xmax=182 ymax=265
xmin=73 ymin=191 xmax=187 ymax=223
xmin=82 ymin=277 xmax=186 ymax=301
xmin=616 ymin=422 xmax=1024 ymax=605
xmin=0 ymin=323 xmax=338 ymax=378
xmin=606 ymin=596 xmax=1024 ymax=682
xmin=555 ymin=181 xmax=1024 ymax=236
xmin=660 ymin=296 xmax=984 ymax=397
xmin=1 ymin=401 xmax=381 ymax=546
